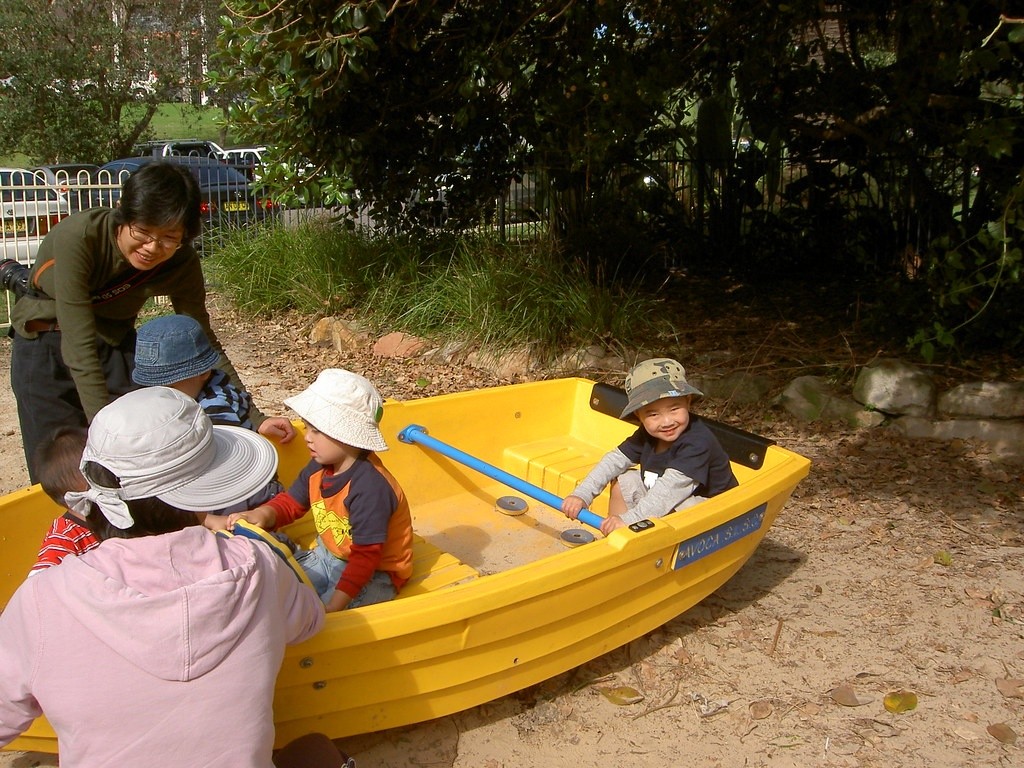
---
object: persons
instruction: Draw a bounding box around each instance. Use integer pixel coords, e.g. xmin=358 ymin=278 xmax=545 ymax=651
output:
xmin=227 ymin=369 xmax=414 ymax=613
xmin=0 ymin=386 xmax=325 ymax=768
xmin=562 ymin=358 xmax=739 ymax=536
xmin=27 ymin=425 xmax=101 ymax=578
xmin=132 ymin=315 xmax=296 ymax=555
xmin=7 ymin=162 xmax=298 ymax=529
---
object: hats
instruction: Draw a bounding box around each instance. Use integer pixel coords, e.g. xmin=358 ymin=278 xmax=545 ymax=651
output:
xmin=283 ymin=368 xmax=389 ymax=451
xmin=64 ymin=385 xmax=279 ymax=530
xmin=619 ymin=358 xmax=704 ymax=420
xmin=131 ymin=315 xmax=221 ymax=386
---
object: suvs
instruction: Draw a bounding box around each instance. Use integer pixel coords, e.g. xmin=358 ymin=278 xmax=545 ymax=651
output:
xmin=129 ymin=139 xmax=225 ymax=162
xmin=218 ymin=147 xmax=327 ymax=206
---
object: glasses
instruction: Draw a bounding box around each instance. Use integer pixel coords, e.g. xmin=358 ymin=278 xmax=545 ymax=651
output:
xmin=128 ymin=219 xmax=187 ymax=250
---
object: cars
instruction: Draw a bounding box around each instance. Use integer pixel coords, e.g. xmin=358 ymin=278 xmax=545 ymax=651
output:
xmin=0 ymin=168 xmax=73 ymax=265
xmin=65 ymin=156 xmax=285 ymax=256
xmin=30 ymin=164 xmax=101 ymax=199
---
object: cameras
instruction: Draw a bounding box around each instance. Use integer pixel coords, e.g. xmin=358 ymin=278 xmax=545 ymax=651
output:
xmin=0 ymin=258 xmax=32 ymax=305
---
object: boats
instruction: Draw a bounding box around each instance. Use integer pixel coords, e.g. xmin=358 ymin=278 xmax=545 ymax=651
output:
xmin=0 ymin=379 xmax=811 ymax=752
